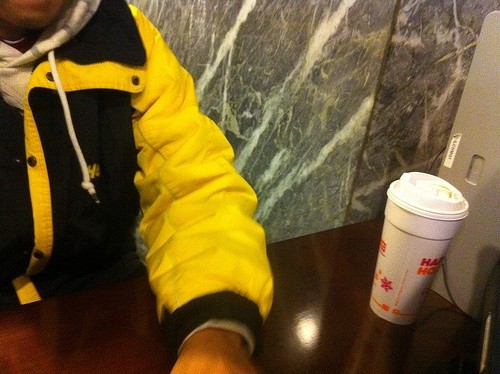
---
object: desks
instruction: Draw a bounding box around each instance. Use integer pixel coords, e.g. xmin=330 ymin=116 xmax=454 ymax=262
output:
xmin=0 ymin=215 xmax=500 ymax=373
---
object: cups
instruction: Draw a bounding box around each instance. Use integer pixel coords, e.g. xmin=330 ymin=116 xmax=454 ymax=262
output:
xmin=369 ymin=172 xmax=470 ymax=325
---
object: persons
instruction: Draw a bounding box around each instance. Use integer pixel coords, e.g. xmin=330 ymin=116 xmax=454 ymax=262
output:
xmin=2 ymin=0 xmax=276 ymax=373
